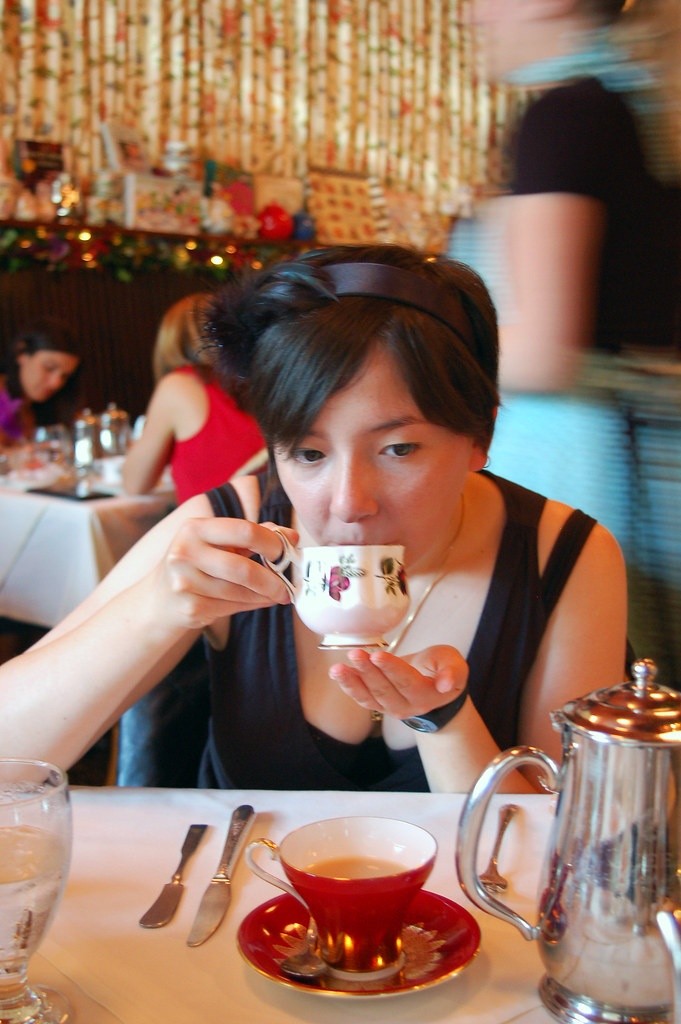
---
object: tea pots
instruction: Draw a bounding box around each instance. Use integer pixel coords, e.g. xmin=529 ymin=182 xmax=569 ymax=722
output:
xmin=453 ymin=657 xmax=681 ymax=1024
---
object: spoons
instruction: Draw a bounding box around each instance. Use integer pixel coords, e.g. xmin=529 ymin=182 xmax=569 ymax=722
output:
xmin=281 ymin=915 xmax=329 ymax=978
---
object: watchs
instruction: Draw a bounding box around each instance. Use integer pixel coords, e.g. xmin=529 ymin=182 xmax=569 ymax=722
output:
xmin=399 ymin=684 xmax=467 ymax=733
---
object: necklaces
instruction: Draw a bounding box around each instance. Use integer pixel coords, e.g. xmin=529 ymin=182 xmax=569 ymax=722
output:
xmin=364 ymin=489 xmax=467 ymax=722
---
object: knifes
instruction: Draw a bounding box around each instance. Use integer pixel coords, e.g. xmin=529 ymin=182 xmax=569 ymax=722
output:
xmin=140 ymin=824 xmax=209 ymax=928
xmin=184 ymin=804 xmax=256 ymax=948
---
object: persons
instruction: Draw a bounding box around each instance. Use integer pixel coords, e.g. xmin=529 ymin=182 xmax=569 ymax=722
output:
xmin=441 ymin=0 xmax=654 ymax=666
xmin=0 ymin=242 xmax=628 ymax=796
xmin=0 ymin=324 xmax=87 ymax=450
xmin=118 ymin=289 xmax=269 ymax=509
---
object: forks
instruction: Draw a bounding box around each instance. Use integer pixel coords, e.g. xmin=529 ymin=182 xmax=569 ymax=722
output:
xmin=475 ymin=805 xmax=520 ymax=895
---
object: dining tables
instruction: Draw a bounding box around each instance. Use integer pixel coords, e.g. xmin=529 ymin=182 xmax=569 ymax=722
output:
xmin=3 ymin=440 xmax=181 ymax=632
xmin=0 ymin=784 xmax=559 ymax=1023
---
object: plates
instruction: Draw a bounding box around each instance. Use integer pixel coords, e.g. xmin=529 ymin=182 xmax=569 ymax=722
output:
xmin=235 ymin=886 xmax=482 ymax=1000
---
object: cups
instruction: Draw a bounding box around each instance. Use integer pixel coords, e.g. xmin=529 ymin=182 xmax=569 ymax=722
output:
xmin=0 ymin=756 xmax=72 ymax=1024
xmin=261 ymin=528 xmax=410 ymax=651
xmin=244 ymin=815 xmax=439 ymax=982
